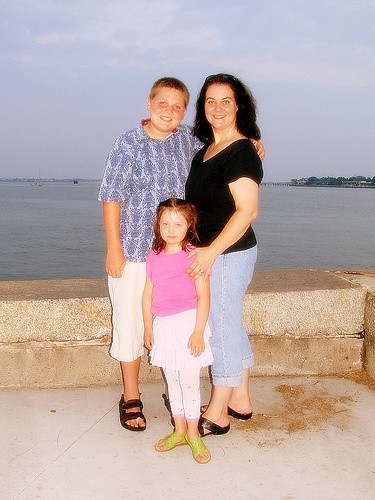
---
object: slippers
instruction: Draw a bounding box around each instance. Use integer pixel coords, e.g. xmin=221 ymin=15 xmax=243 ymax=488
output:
xmin=201 ymin=403 xmax=253 ymax=420
xmin=197 ymin=415 xmax=230 ymax=437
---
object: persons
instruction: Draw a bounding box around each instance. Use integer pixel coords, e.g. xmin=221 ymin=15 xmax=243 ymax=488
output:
xmin=95 ymin=76 xmax=267 ymax=432
xmin=185 ymin=72 xmax=265 ymax=438
xmin=142 ymin=197 xmax=214 ymax=466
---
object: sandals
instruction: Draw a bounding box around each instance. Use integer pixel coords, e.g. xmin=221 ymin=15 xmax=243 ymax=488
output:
xmin=119 ymin=395 xmax=147 ymax=432
xmin=155 ymin=432 xmax=188 ymax=452
xmin=185 ymin=434 xmax=211 ymax=464
xmin=162 ymin=394 xmax=175 ymax=426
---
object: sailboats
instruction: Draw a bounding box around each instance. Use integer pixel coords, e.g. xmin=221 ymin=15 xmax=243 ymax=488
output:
xmin=31 ymin=167 xmax=43 ymax=186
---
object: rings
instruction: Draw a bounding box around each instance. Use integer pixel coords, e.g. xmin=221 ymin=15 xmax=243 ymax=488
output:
xmin=200 ymin=270 xmax=203 ymax=273
